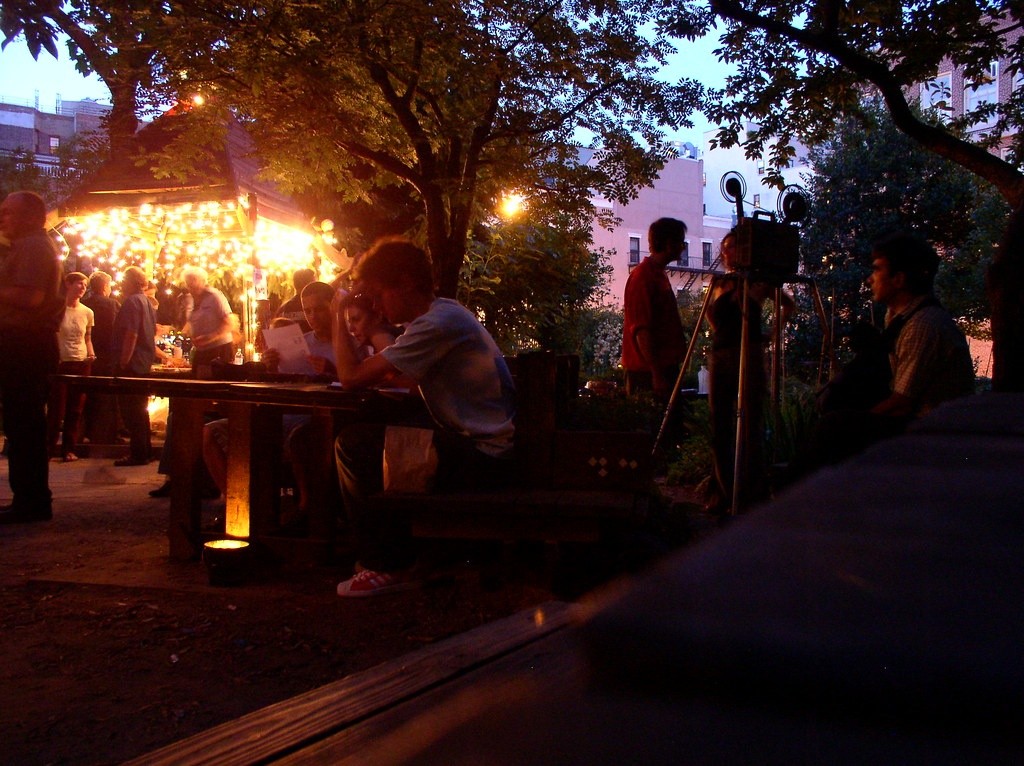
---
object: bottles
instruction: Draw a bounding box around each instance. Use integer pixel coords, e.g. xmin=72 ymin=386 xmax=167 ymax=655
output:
xmin=234 ymin=348 xmax=244 ymax=365
xmin=156 ymin=330 xmax=197 ymax=365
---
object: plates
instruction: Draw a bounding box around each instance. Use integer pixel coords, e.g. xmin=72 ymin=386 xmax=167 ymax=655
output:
xmin=151 ymin=366 xmax=193 ymax=373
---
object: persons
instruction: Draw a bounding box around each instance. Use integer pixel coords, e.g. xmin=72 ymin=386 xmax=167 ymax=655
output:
xmin=275 ymin=269 xmax=316 ymax=332
xmin=280 ymin=290 xmax=418 ymax=540
xmin=847 ymin=319 xmax=879 ymax=352
xmin=179 ymin=266 xmax=237 ymax=380
xmin=621 ymin=217 xmax=690 ymax=501
xmin=46 ymin=273 xmax=96 ymax=462
xmin=147 ymin=317 xmax=303 ymax=498
xmin=808 ymin=233 xmax=976 ymax=480
xmin=0 ymin=191 xmax=68 ymax=525
xmin=198 ymin=281 xmax=370 ymax=535
xmin=329 ymin=236 xmax=525 ymax=597
xmin=82 ymin=271 xmax=131 ymax=445
xmin=112 ymin=266 xmax=156 ymax=465
xmin=705 ymin=230 xmax=796 ymax=516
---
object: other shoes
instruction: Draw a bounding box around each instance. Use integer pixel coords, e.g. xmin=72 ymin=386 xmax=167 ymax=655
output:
xmin=0 ymin=504 xmax=52 ymax=524
xmin=198 ymin=487 xmax=223 ymax=504
xmin=336 ymin=568 xmax=407 ymax=598
xmin=263 ymin=507 xmax=312 ymax=538
xmin=147 ymin=481 xmax=175 ymax=498
xmin=113 ymin=452 xmax=157 ymax=467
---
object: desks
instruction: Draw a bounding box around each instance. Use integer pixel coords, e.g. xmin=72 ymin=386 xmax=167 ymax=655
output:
xmin=56 ymin=372 xmax=422 ymax=590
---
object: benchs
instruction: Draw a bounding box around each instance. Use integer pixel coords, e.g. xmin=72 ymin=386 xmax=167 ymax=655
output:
xmin=376 ymin=351 xmax=672 ymax=593
xmin=114 ymin=600 xmax=576 ymax=766
